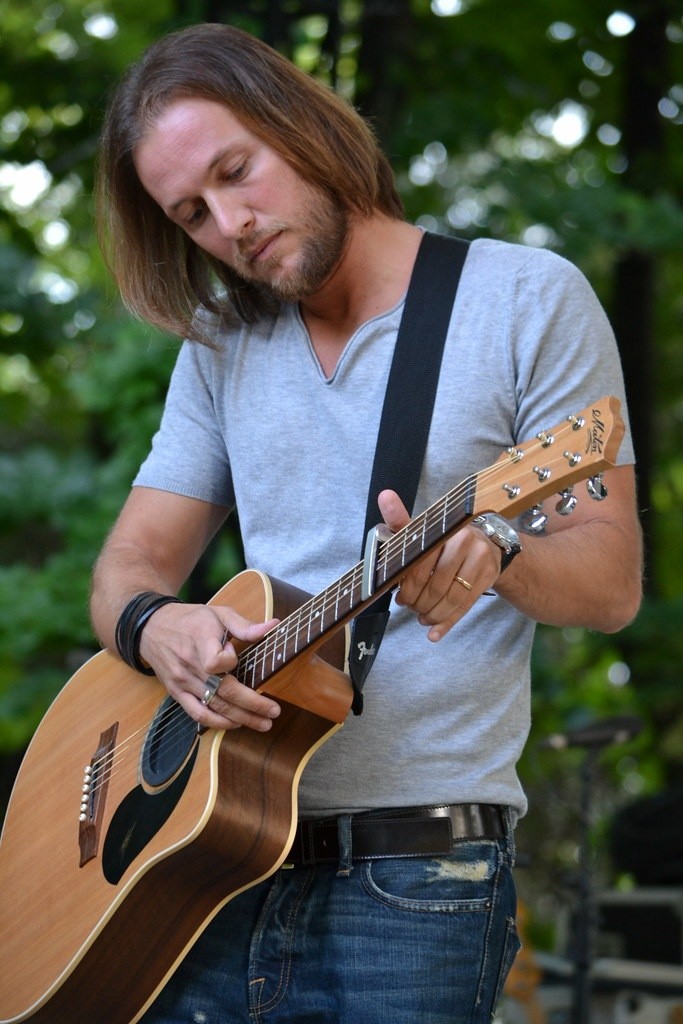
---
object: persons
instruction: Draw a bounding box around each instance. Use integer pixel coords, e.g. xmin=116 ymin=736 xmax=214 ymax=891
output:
xmin=91 ymin=22 xmax=642 ymax=1024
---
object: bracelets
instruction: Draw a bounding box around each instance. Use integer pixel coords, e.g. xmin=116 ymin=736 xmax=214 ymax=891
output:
xmin=115 ymin=591 xmax=181 ymax=676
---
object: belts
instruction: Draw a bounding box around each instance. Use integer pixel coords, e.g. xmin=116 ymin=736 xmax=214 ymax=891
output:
xmin=280 ymin=805 xmax=505 ymax=870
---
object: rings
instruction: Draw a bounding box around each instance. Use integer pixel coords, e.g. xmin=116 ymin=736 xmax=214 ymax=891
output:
xmin=202 ymin=691 xmax=215 ymax=705
xmin=205 ymin=671 xmax=226 ymax=693
xmin=455 ymin=576 xmax=472 ymax=590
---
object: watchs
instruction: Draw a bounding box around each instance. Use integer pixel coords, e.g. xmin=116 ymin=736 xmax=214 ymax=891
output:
xmin=474 ymin=515 xmax=522 ymax=574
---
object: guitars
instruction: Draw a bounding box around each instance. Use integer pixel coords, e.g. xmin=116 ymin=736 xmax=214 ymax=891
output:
xmin=0 ymin=395 xmax=627 ymax=1023
